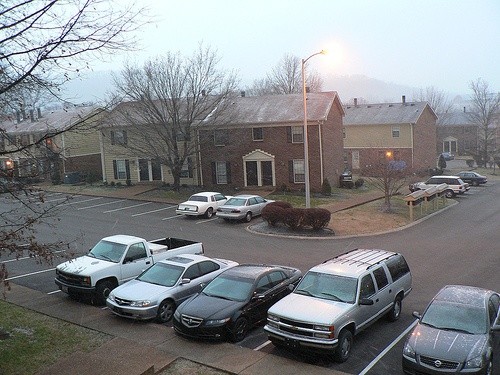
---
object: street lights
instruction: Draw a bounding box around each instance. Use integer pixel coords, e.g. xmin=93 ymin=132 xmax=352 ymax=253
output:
xmin=301 ymin=48 xmax=330 ymax=209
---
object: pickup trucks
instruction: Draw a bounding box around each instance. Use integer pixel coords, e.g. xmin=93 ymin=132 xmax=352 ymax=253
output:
xmin=54 ymin=233 xmax=205 ymax=305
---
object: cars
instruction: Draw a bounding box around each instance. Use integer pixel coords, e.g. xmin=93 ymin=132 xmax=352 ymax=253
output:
xmin=439 ymin=151 xmax=456 ymax=160
xmin=175 ymin=192 xmax=234 ymax=219
xmin=402 ymin=284 xmax=500 ymax=375
xmin=408 ymin=172 xmax=489 ymax=199
xmin=173 ymin=263 xmax=303 ymax=344
xmin=216 ymin=195 xmax=276 ymax=223
xmin=105 ymin=253 xmax=240 ymax=324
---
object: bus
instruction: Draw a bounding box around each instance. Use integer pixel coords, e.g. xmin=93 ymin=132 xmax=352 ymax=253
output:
xmin=0 ymin=157 xmax=16 ymax=192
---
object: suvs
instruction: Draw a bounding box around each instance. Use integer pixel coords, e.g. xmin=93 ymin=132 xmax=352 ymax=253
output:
xmin=263 ymin=247 xmax=413 ymax=363
